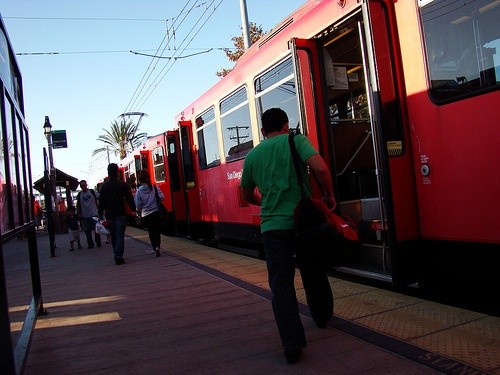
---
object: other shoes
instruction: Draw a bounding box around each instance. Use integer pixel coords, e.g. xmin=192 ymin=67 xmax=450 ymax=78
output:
xmin=152 ymin=246 xmax=162 ymax=257
xmin=283 ymin=348 xmax=303 ymax=364
xmin=314 ymin=320 xmax=329 ymax=328
xmin=87 ymin=244 xmax=94 ymax=249
xmin=114 ymin=255 xmax=126 ymax=265
xmin=96 ymin=243 xmax=102 ymax=247
xmin=105 ymin=240 xmax=112 ymax=244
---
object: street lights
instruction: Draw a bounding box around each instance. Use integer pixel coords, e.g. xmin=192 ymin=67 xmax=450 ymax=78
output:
xmin=43 ymin=116 xmax=54 ymax=170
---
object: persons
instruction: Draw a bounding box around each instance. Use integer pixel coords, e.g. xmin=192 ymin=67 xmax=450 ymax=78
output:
xmin=77 ymin=180 xmax=102 ymax=249
xmin=241 ymin=108 xmax=337 ymax=365
xmin=65 ymin=206 xmax=82 ymax=251
xmin=97 ymin=163 xmax=136 ymax=265
xmin=134 ymin=169 xmax=165 ymax=257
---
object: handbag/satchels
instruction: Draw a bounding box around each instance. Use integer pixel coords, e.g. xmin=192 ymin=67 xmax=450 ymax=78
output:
xmin=288 ymin=122 xmax=365 ymax=267
xmin=153 ymin=186 xmax=167 ymax=223
xmin=93 ymin=217 xmax=110 ymax=235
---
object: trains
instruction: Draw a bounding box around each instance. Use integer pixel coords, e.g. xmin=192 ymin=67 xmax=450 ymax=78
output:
xmin=96 ymin=0 xmax=500 ymax=283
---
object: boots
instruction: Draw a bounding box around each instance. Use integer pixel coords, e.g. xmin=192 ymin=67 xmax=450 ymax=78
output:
xmin=77 ymin=240 xmax=83 ymax=249
xmin=68 ymin=240 xmax=75 ymax=251
